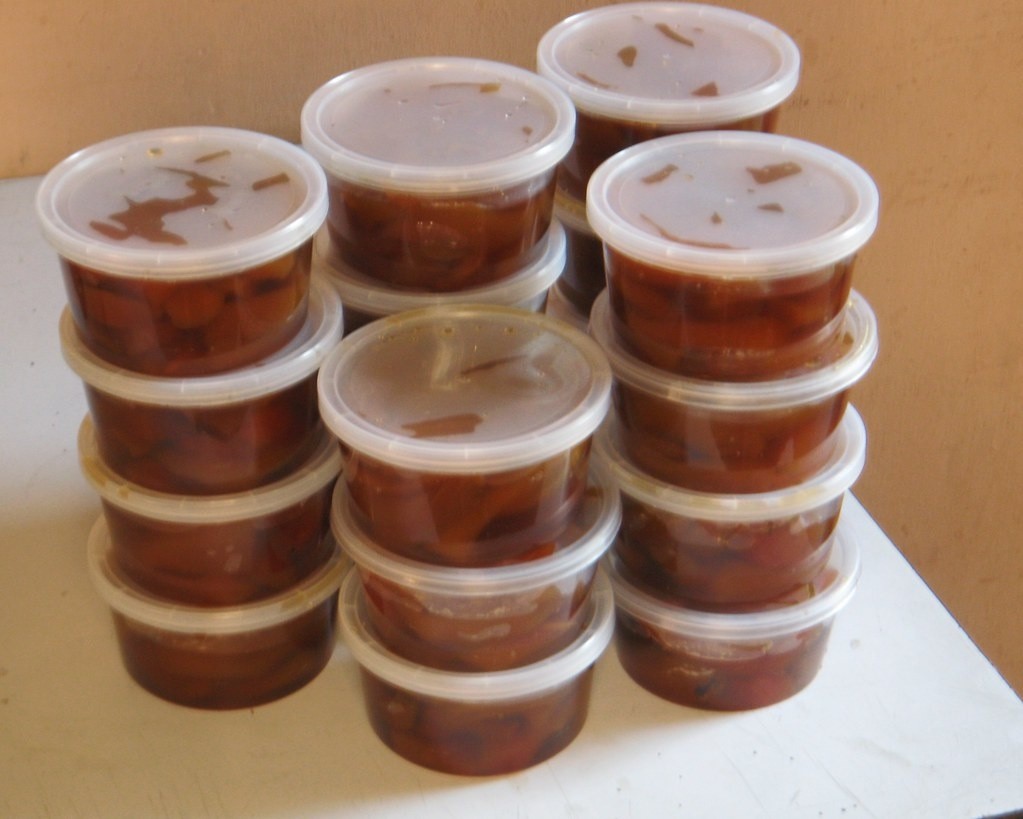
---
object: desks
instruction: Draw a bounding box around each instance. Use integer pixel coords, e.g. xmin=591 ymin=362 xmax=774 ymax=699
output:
xmin=0 ymin=176 xmax=1023 ymax=819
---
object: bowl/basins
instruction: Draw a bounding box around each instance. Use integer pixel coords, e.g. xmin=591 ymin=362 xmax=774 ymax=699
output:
xmin=35 ymin=3 xmax=882 ymax=769
